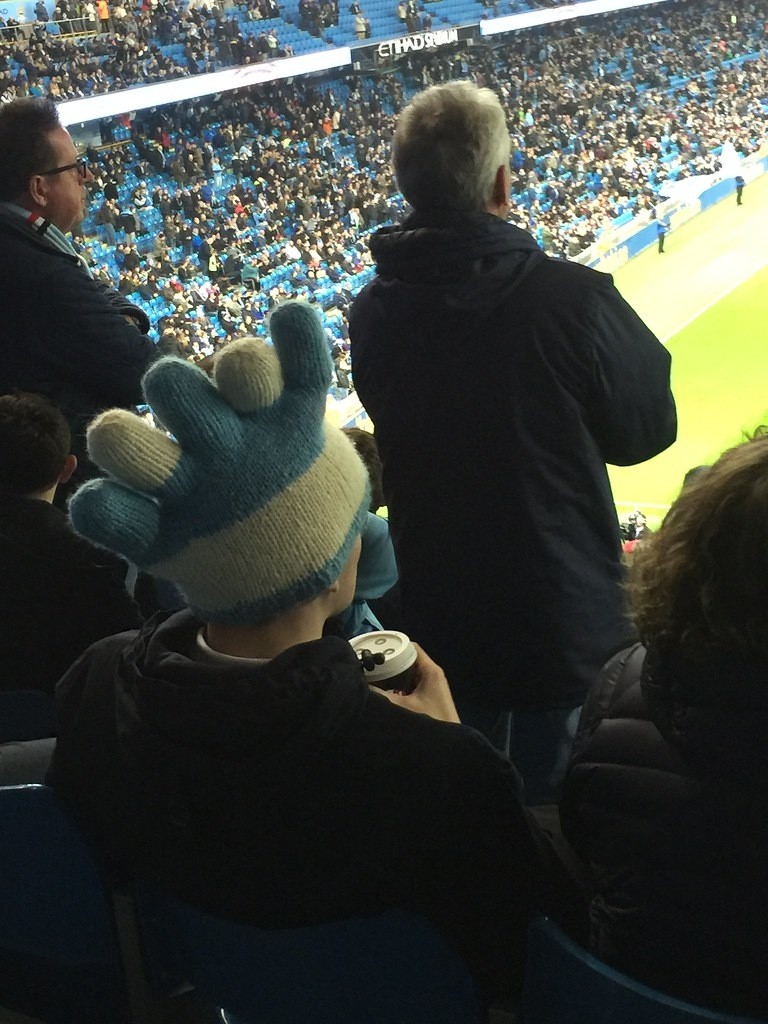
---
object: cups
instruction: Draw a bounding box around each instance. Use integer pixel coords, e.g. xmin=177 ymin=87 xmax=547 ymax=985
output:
xmin=349 ymin=631 xmax=418 ymax=696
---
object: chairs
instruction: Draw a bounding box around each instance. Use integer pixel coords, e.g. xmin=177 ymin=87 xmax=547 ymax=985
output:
xmin=0 ymin=0 xmax=768 ymax=393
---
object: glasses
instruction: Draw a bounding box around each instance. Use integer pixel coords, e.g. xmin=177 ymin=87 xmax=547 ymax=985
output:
xmin=26 ymin=158 xmax=87 ymax=189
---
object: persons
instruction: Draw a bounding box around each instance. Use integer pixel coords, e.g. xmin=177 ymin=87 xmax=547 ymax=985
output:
xmin=1 ymin=1 xmax=766 ymax=1024
xmin=0 ymin=99 xmax=167 ymax=498
xmin=0 ymin=392 xmax=87 ymax=555
xmin=349 ymin=79 xmax=678 ymax=724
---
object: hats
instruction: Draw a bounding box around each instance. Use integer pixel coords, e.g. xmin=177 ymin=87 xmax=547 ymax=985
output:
xmin=69 ymin=302 xmax=373 ymax=631
